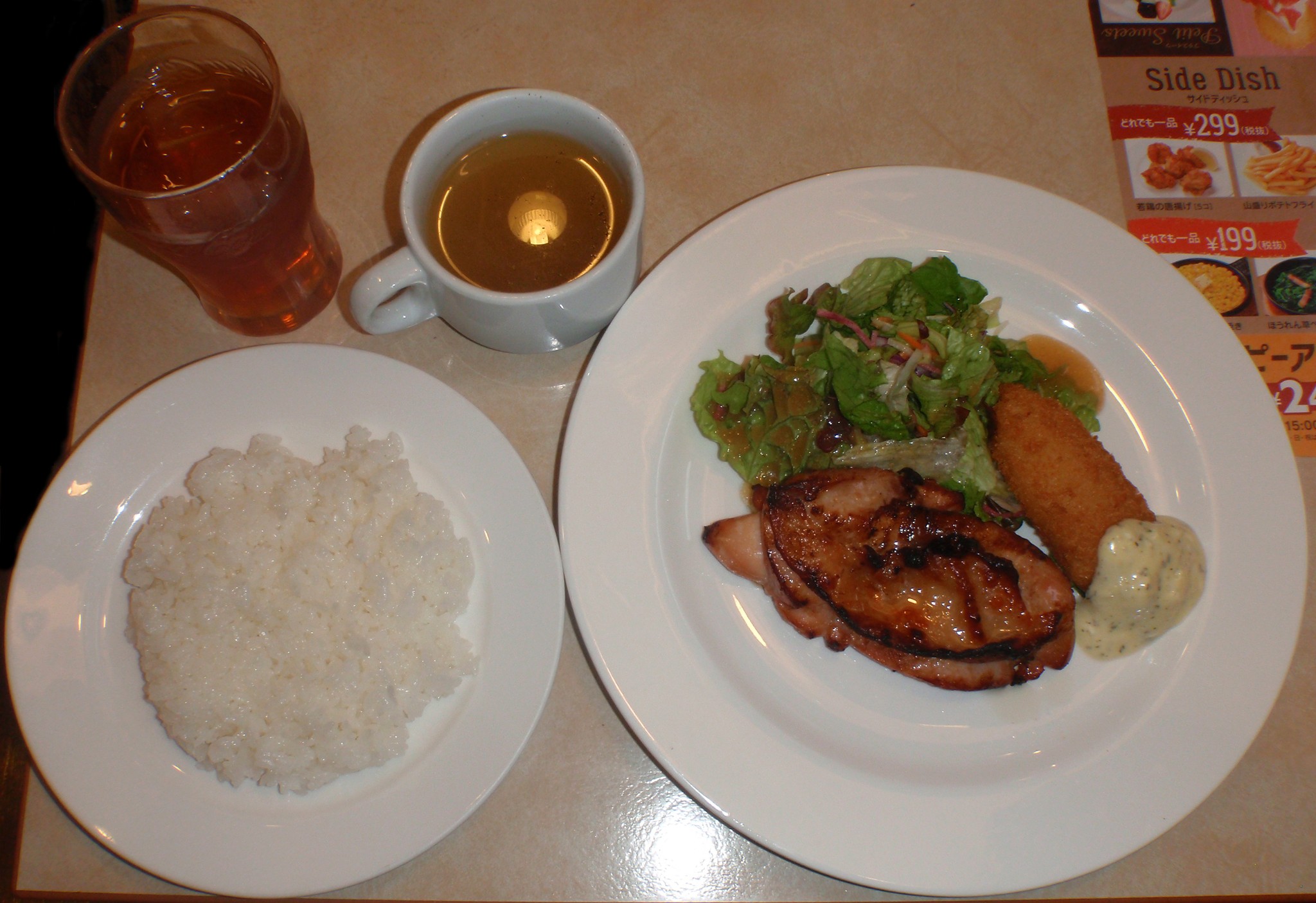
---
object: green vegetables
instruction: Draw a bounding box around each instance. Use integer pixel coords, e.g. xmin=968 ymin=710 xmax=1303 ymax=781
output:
xmin=1274 ymin=268 xmax=1316 ymax=313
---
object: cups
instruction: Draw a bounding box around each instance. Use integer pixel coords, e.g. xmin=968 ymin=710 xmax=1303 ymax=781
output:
xmin=58 ymin=1 xmax=343 ymax=339
xmin=350 ymin=86 xmax=645 ymax=357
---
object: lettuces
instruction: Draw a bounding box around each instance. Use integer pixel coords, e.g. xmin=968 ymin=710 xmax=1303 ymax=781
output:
xmin=687 ymin=253 xmax=1100 ymax=526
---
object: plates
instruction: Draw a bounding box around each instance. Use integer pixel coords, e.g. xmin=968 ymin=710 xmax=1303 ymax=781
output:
xmin=3 ymin=342 xmax=561 ymax=902
xmin=557 ymin=161 xmax=1312 ymax=903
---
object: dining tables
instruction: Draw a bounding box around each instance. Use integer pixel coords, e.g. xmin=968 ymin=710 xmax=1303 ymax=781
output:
xmin=0 ymin=0 xmax=1316 ymax=903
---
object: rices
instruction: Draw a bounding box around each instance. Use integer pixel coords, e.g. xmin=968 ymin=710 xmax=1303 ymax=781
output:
xmin=124 ymin=425 xmax=482 ymax=791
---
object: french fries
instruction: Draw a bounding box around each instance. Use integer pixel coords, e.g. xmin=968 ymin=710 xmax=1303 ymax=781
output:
xmin=1242 ymin=135 xmax=1316 ymax=194
